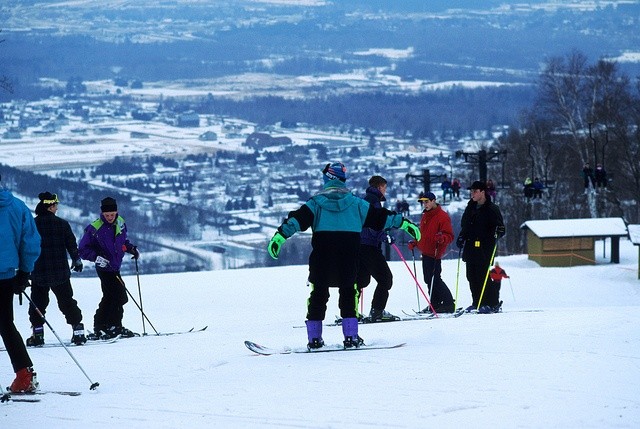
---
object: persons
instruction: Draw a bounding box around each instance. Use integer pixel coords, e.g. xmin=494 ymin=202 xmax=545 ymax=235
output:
xmin=26 ymin=192 xmax=86 ymax=346
xmin=407 ymin=192 xmax=455 ymax=313
xmin=268 ymin=161 xmax=422 ymax=354
xmin=489 ymin=261 xmax=510 ymax=293
xmin=0 ymin=173 xmax=42 ymax=393
xmin=456 ymin=180 xmax=506 ymax=313
xmin=78 ymin=196 xmax=141 ymax=338
xmin=357 ymin=176 xmax=401 ymax=323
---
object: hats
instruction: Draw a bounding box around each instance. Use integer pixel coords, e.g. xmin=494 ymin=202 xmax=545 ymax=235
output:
xmin=323 ymin=161 xmax=347 ymax=184
xmin=467 ymin=180 xmax=487 ymax=190
xmin=39 ymin=191 xmax=60 ymax=204
xmin=100 ymin=196 xmax=118 ymax=211
xmin=418 ymin=191 xmax=435 ymax=201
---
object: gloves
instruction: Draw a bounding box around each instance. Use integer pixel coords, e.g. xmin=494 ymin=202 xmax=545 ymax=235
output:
xmin=71 ymin=259 xmax=82 ymax=271
xmin=399 ymin=219 xmax=421 ymax=242
xmin=14 ymin=271 xmax=32 ymax=295
xmin=94 ymin=255 xmax=109 ymax=268
xmin=456 ymin=237 xmax=465 ymax=248
xmin=267 ymin=232 xmax=286 ymax=260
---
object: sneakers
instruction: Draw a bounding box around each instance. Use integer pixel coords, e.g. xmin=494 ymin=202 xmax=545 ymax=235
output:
xmin=466 ymin=305 xmax=477 ymax=312
xmin=308 ymin=337 xmax=324 ymax=349
xmin=71 ymin=334 xmax=86 ymax=345
xmin=436 ymin=303 xmax=455 ymax=313
xmin=422 ymin=303 xmax=442 ymax=313
xmin=369 ymin=308 xmax=400 ymax=322
xmin=26 ymin=336 xmax=45 ymax=346
xmin=477 ymin=305 xmax=501 ymax=313
xmin=343 ymin=335 xmax=364 ymax=349
xmin=94 ymin=330 xmax=116 ymax=339
xmin=119 ymin=327 xmax=135 ymax=337
xmin=9 ymin=368 xmax=38 ymax=392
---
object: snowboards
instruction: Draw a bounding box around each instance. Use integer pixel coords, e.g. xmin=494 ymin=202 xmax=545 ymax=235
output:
xmin=246 ymin=340 xmax=406 ymax=356
xmin=1 ymin=333 xmax=121 ymax=350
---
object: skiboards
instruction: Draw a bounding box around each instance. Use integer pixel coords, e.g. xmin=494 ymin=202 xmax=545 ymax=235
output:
xmin=403 ymin=307 xmax=541 ymax=318
xmin=294 ymin=307 xmax=467 ymax=329
xmin=84 ymin=325 xmax=208 ymax=340
xmin=1 ymin=389 xmax=81 ymax=403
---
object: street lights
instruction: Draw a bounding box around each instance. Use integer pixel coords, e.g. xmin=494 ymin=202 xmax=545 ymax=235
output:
xmin=456 ymin=150 xmax=498 ymax=182
xmin=406 ymin=168 xmax=441 ymax=192
